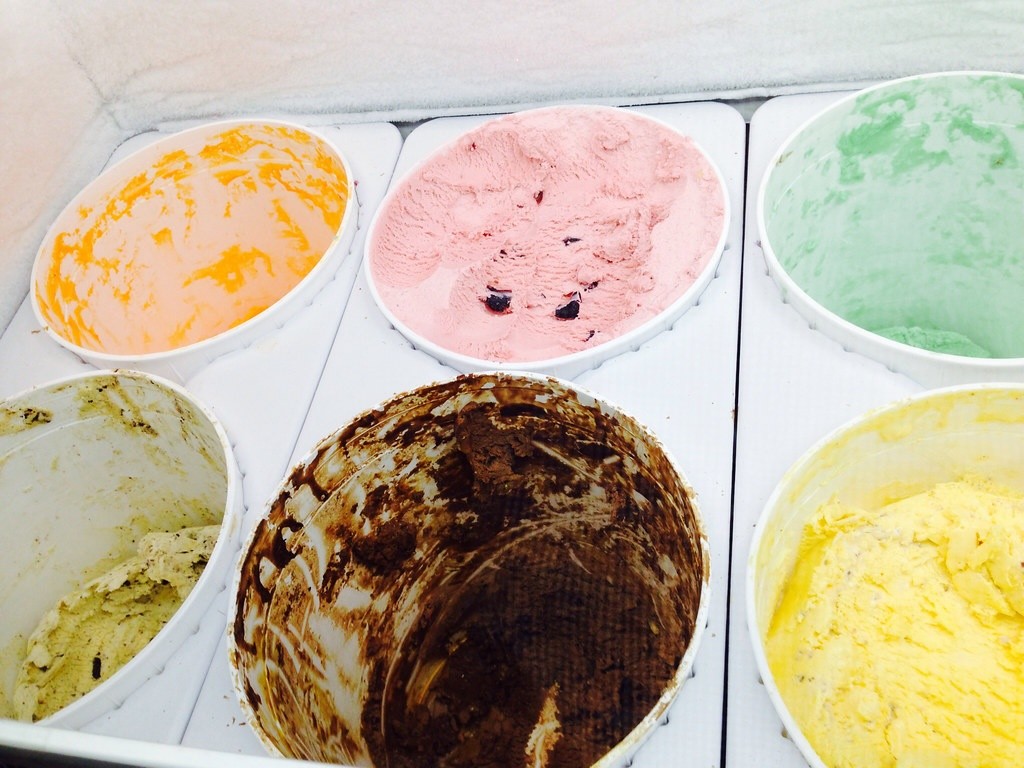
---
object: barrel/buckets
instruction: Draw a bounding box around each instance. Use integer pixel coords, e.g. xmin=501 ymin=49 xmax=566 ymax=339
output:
xmin=29 ymin=119 xmax=360 ymax=386
xmin=743 ymin=381 xmax=1024 ymax=768
xmin=0 ymin=368 xmax=245 ymax=731
xmin=755 ymin=70 xmax=1024 ymax=390
xmin=364 ymin=104 xmax=732 ymax=383
xmin=227 ymin=368 xmax=715 ymax=768
